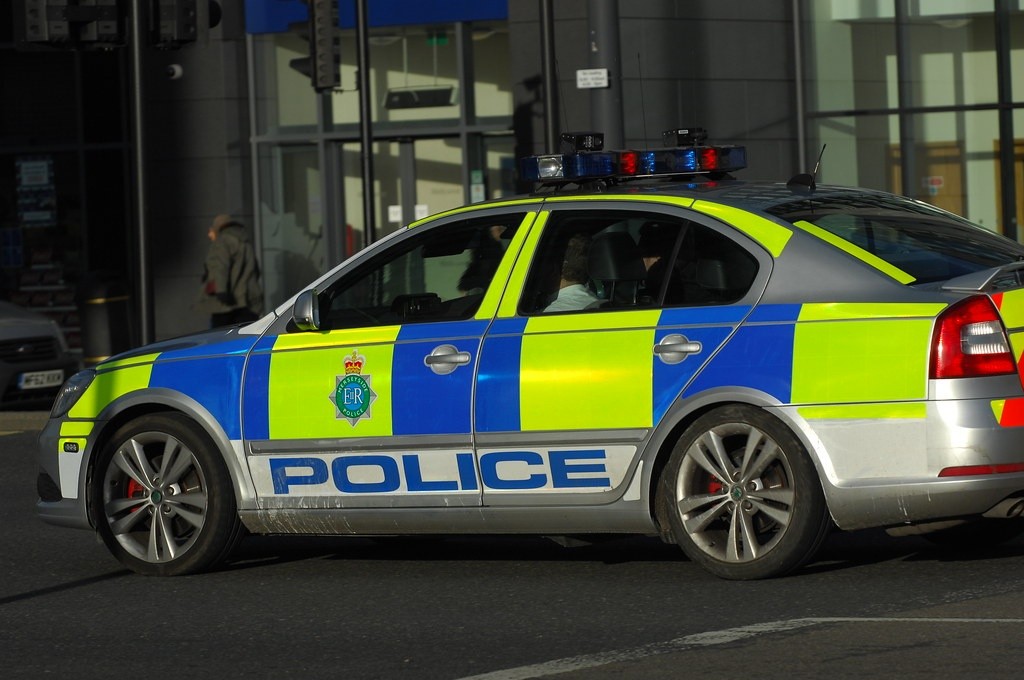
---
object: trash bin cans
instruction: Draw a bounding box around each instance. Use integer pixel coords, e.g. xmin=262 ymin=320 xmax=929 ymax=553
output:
xmin=79 ymin=288 xmax=141 ymax=368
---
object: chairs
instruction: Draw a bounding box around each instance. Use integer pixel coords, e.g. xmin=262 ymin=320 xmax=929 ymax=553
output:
xmin=583 ymin=230 xmax=647 ymax=312
xmin=639 ymin=221 xmax=683 ymax=305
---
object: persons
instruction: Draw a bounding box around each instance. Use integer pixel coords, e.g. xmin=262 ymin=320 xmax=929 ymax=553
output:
xmin=458 ymin=223 xmax=662 ymax=312
xmin=196 ymin=212 xmax=264 ymax=331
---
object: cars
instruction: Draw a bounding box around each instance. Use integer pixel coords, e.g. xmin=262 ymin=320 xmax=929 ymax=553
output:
xmin=0 ymin=303 xmax=82 ymax=412
xmin=17 ymin=129 xmax=1024 ymax=580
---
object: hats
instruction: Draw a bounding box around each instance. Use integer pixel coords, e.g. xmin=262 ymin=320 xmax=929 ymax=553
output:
xmin=212 ymin=213 xmax=245 ymax=232
